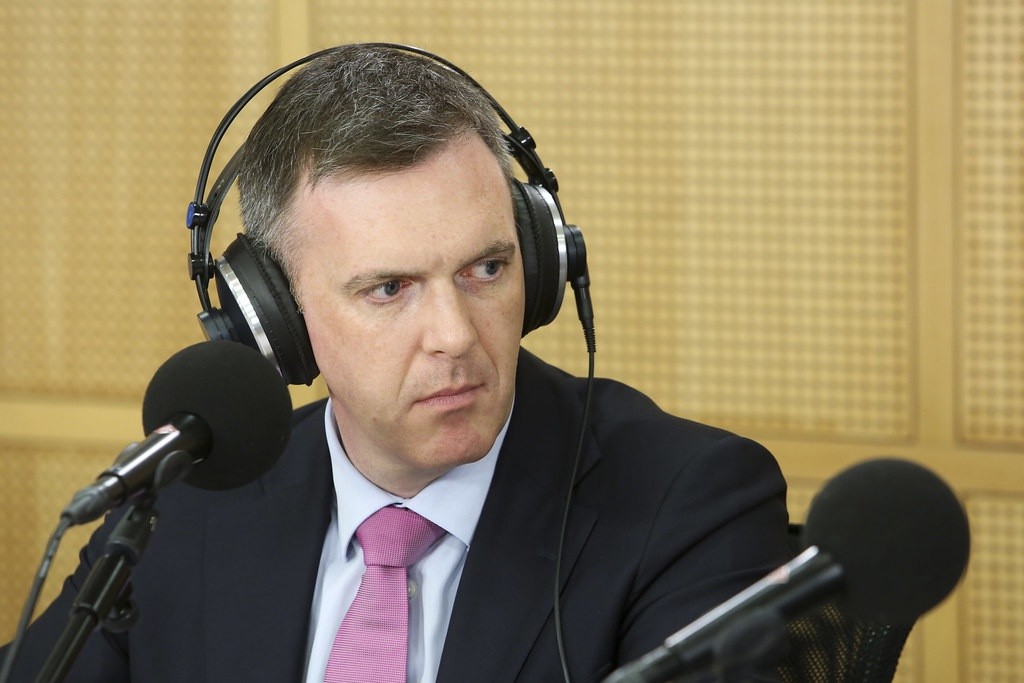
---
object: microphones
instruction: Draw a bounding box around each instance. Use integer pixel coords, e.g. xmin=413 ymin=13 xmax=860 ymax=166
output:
xmin=601 ymin=457 xmax=971 ymax=683
xmin=61 ymin=339 xmax=296 ymax=527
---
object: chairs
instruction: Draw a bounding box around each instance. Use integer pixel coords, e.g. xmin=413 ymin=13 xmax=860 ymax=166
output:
xmin=775 ymin=523 xmax=917 ymax=682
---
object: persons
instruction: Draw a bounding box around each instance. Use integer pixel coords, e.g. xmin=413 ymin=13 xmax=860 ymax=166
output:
xmin=0 ymin=44 xmax=789 ymax=683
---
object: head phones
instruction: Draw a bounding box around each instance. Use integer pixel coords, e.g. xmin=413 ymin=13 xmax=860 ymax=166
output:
xmin=187 ymin=41 xmax=595 ymax=385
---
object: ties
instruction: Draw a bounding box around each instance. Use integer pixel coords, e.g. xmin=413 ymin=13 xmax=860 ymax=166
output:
xmin=324 ymin=506 xmax=446 ymax=683
